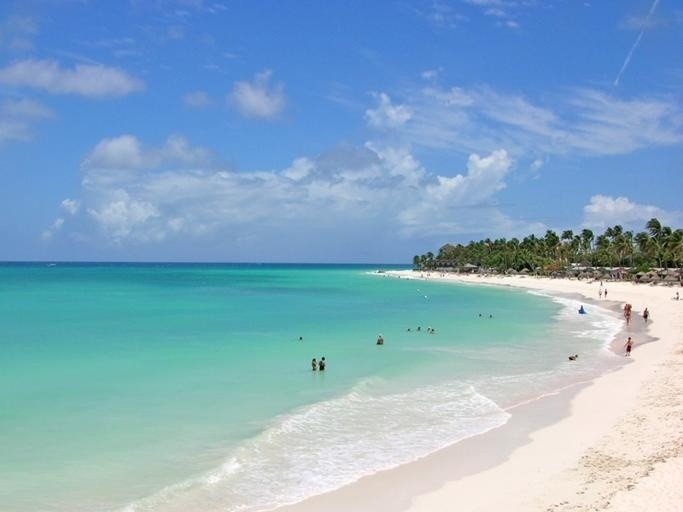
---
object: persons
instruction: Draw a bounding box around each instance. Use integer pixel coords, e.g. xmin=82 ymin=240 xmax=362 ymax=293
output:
xmin=599 ymin=289 xmax=602 ymax=297
xmin=311 ymin=358 xmax=318 ymax=371
xmin=643 ymin=308 xmax=649 ymax=321
xmin=568 ymin=354 xmax=578 ymax=362
xmin=623 ymin=303 xmax=632 ymax=325
xmin=624 ymin=337 xmax=633 ymax=357
xmin=318 ymin=356 xmax=325 ymax=371
xmin=407 ymin=326 xmax=435 ymax=334
xmin=676 ymin=291 xmax=680 ymax=301
xmin=604 ymin=289 xmax=607 ymax=296
xmin=376 ymin=335 xmax=384 ymax=345
